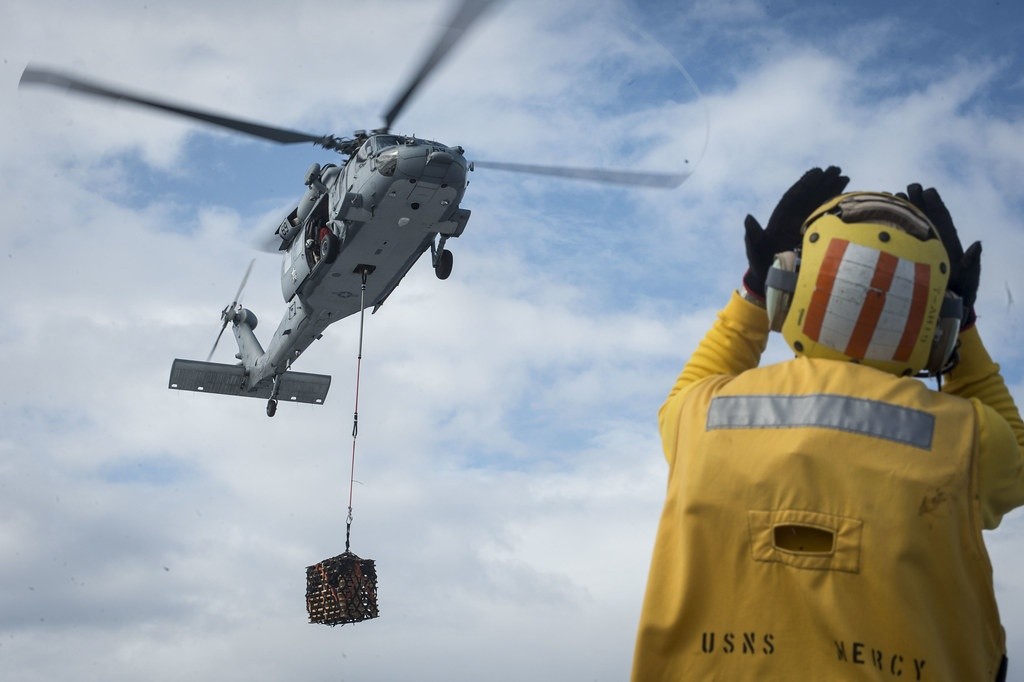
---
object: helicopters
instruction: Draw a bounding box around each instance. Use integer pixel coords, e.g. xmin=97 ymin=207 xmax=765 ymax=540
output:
xmin=20 ymin=1 xmax=692 ymax=416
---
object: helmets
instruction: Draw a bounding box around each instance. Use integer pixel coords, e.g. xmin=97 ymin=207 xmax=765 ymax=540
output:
xmin=781 ymin=192 xmax=950 ymax=376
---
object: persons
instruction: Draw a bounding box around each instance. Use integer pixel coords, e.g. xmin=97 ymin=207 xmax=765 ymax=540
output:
xmin=628 ymin=166 xmax=1024 ymax=681
xmin=306 ymin=239 xmax=321 ymax=262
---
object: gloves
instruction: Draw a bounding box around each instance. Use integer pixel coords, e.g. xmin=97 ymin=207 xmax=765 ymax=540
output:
xmin=895 ymin=183 xmax=982 ymax=332
xmin=743 ymin=166 xmax=850 ymax=301
